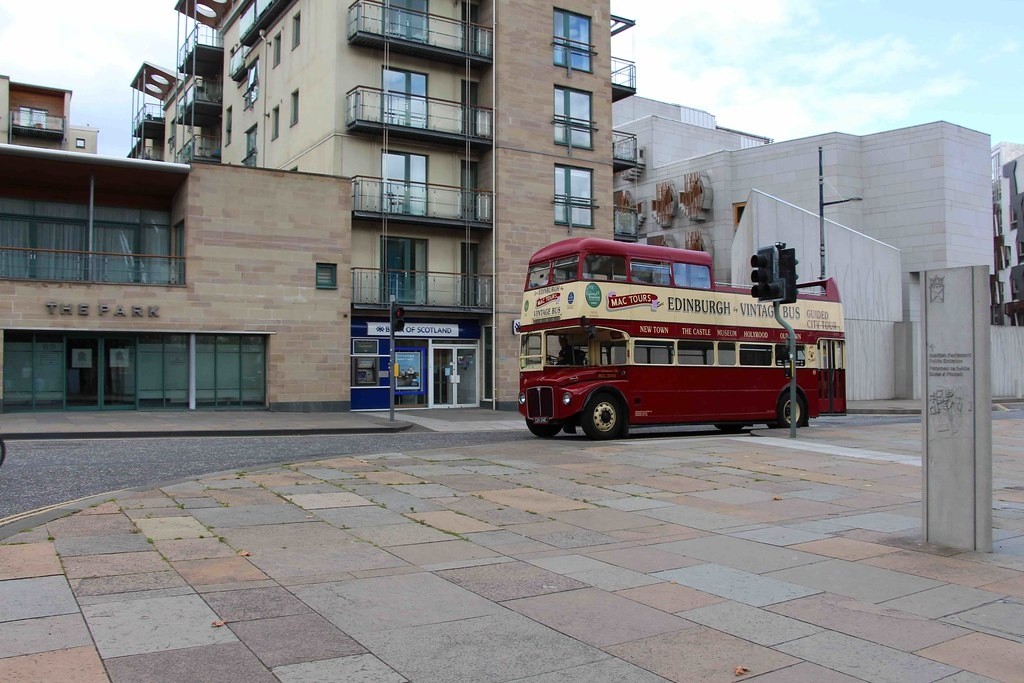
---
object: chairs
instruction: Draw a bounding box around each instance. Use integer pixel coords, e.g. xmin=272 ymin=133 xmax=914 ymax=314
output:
xmin=573 ymin=349 xmax=586 ymax=365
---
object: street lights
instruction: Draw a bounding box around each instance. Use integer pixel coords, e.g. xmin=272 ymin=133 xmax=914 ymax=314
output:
xmin=818 ymin=145 xmax=864 ymax=293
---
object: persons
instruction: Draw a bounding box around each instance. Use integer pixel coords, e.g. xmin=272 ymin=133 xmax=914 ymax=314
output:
xmin=556 ymin=336 xmax=572 ymax=365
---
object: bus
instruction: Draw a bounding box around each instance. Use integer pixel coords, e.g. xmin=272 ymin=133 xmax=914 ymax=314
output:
xmin=517 ymin=237 xmax=846 ymax=442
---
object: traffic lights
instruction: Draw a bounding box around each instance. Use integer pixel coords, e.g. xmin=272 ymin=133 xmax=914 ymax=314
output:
xmin=391 ymin=305 xmax=404 ymax=333
xmin=779 ymin=248 xmax=799 ymax=303
xmin=751 ymin=245 xmax=786 ymax=303
xmin=395 ymin=364 xmax=400 ymax=377
